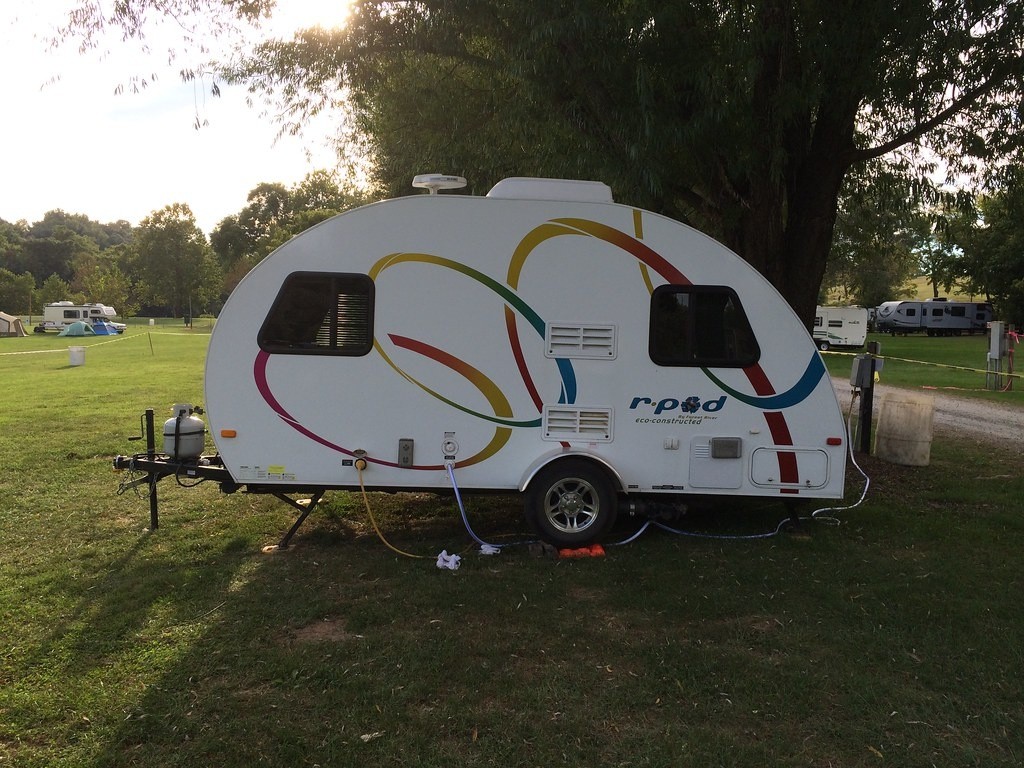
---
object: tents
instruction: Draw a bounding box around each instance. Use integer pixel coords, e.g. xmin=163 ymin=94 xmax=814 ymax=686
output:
xmin=1 ymin=311 xmax=30 ymax=337
xmin=57 ymin=321 xmax=97 ymax=337
xmin=92 ymin=321 xmax=119 ymax=335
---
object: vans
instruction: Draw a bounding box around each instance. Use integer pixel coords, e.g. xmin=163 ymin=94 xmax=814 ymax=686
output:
xmin=812 ymin=296 xmax=992 ymax=352
xmin=44 ymin=301 xmax=117 ymax=331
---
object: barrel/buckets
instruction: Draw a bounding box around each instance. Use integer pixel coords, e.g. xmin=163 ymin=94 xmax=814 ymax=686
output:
xmin=149 ymin=318 xmax=155 ymax=327
